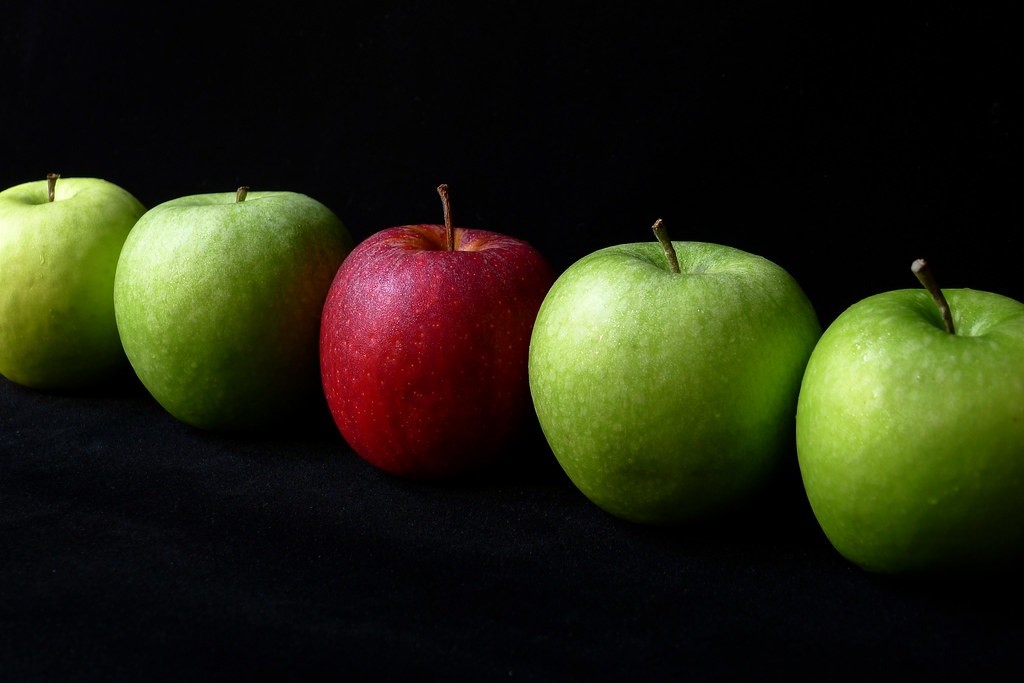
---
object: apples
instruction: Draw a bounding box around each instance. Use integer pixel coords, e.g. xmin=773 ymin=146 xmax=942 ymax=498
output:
xmin=114 ymin=184 xmax=349 ymax=427
xmin=0 ymin=173 xmax=157 ymax=386
xmin=528 ymin=218 xmax=822 ymax=519
xmin=795 ymin=258 xmax=1024 ymax=569
xmin=318 ymin=184 xmax=554 ymax=477
